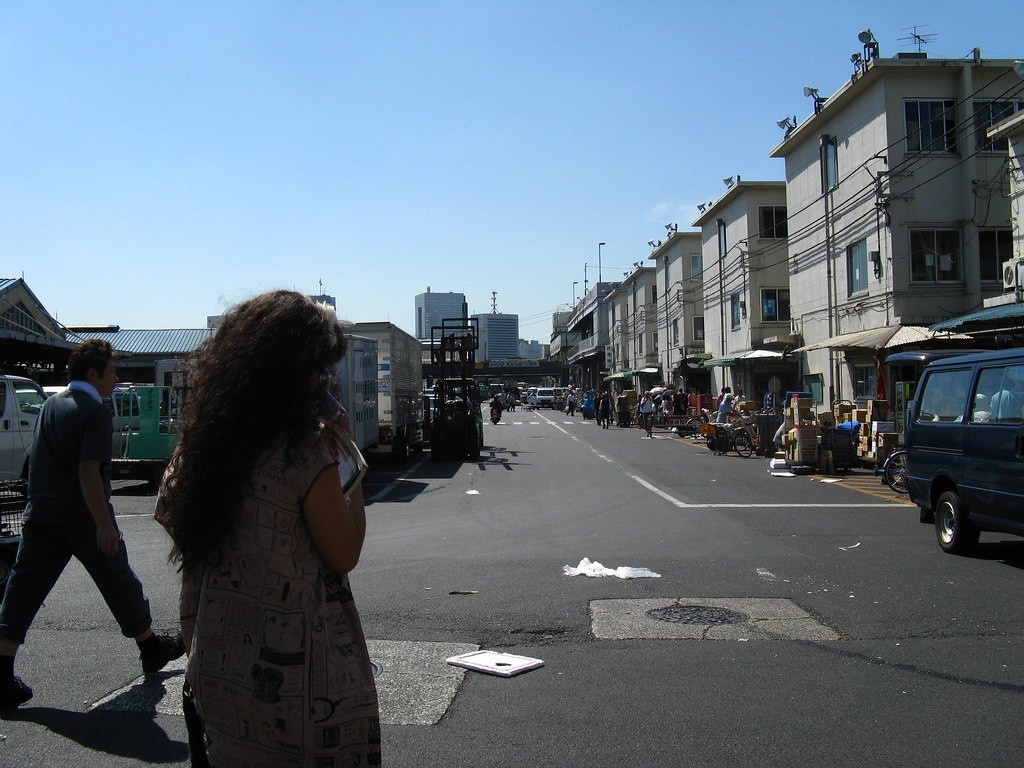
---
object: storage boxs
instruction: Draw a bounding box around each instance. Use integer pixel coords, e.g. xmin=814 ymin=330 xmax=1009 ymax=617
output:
xmin=622 ymin=389 xmax=637 ymax=409
xmin=772 ymin=397 xmax=900 ymax=476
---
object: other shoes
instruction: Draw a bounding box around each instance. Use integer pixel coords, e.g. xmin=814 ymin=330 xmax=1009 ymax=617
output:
xmin=571 ymin=414 xmax=574 ymax=416
xmin=566 ymin=412 xmax=568 ymax=415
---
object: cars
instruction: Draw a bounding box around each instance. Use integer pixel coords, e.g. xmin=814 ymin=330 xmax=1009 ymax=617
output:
xmin=419 ymin=383 xmax=489 ymax=420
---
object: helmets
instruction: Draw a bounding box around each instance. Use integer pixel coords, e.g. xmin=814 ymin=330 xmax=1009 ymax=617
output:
xmin=494 ymin=395 xmax=498 ymax=399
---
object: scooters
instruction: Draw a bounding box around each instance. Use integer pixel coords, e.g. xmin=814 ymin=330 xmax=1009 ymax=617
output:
xmin=490 ymin=405 xmax=501 ymax=424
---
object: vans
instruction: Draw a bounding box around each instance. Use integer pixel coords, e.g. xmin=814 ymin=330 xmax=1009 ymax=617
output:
xmin=0 ymin=375 xmax=55 ymax=497
xmin=536 ymin=389 xmax=555 ymax=411
xmin=880 ymin=349 xmax=1024 ymax=556
xmin=10 ymin=385 xmax=142 ymax=478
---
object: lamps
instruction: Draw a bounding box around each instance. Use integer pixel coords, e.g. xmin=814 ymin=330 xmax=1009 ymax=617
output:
xmin=648 ymin=222 xmax=677 ymax=247
xmin=722 ymin=176 xmax=735 ymax=189
xmin=776 ymin=115 xmax=796 ymax=135
xmin=697 ymin=202 xmax=707 ymax=214
xmin=804 ymin=86 xmax=828 ymax=113
xmin=622 ymin=261 xmax=641 ymax=278
xmin=847 ymin=28 xmax=879 ymax=80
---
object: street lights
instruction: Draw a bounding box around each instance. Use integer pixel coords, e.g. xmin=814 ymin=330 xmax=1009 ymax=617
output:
xmin=598 ymin=243 xmax=606 ymax=283
xmin=556 ymin=303 xmax=569 ymax=313
xmin=573 ymin=281 xmax=578 ymax=310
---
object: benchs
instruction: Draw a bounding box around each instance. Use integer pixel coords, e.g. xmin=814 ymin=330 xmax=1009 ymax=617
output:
xmin=644 ymin=413 xmax=706 ymax=439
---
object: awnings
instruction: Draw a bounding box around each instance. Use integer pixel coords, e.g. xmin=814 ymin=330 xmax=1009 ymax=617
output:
xmin=789 ymin=324 xmax=970 ymax=356
xmin=698 ymin=348 xmax=753 ymax=369
xmin=622 ymin=366 xmax=658 ymax=375
xmin=929 ymin=301 xmax=1024 ymax=332
xmin=603 ymin=370 xmax=628 ymax=380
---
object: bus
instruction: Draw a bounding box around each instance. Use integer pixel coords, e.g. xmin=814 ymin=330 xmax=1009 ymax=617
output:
xmin=489 ymin=381 xmax=527 ymax=396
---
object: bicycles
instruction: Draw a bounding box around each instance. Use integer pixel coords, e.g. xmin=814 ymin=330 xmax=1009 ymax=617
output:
xmin=626 ymin=405 xmax=759 ymax=458
xmin=882 ymin=445 xmax=911 ymax=501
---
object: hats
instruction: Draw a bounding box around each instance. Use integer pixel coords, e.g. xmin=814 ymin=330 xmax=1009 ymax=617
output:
xmin=726 ymin=393 xmax=733 ymax=399
xmin=738 ymin=401 xmax=746 ymax=405
xmin=645 ymin=390 xmax=650 ymax=394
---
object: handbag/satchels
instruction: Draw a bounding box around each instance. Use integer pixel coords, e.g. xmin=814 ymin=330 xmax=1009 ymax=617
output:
xmin=183 ymin=681 xmax=212 ymax=768
xmin=565 ymin=405 xmax=569 ymax=412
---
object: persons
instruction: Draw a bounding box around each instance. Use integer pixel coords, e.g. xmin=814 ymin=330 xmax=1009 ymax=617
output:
xmin=0 ymin=338 xmax=186 ymax=705
xmin=490 ymin=384 xmax=749 ymax=455
xmin=566 ymin=388 xmax=575 ymax=416
xmin=153 ymin=291 xmax=382 ymax=768
xmin=989 ymin=376 xmax=1020 ymax=419
xmin=640 ymin=389 xmax=653 ymax=437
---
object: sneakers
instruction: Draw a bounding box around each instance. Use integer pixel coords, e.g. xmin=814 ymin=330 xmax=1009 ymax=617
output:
xmin=141 ymin=632 xmax=186 ymax=673
xmin=0 ymin=674 xmax=33 ymax=700
